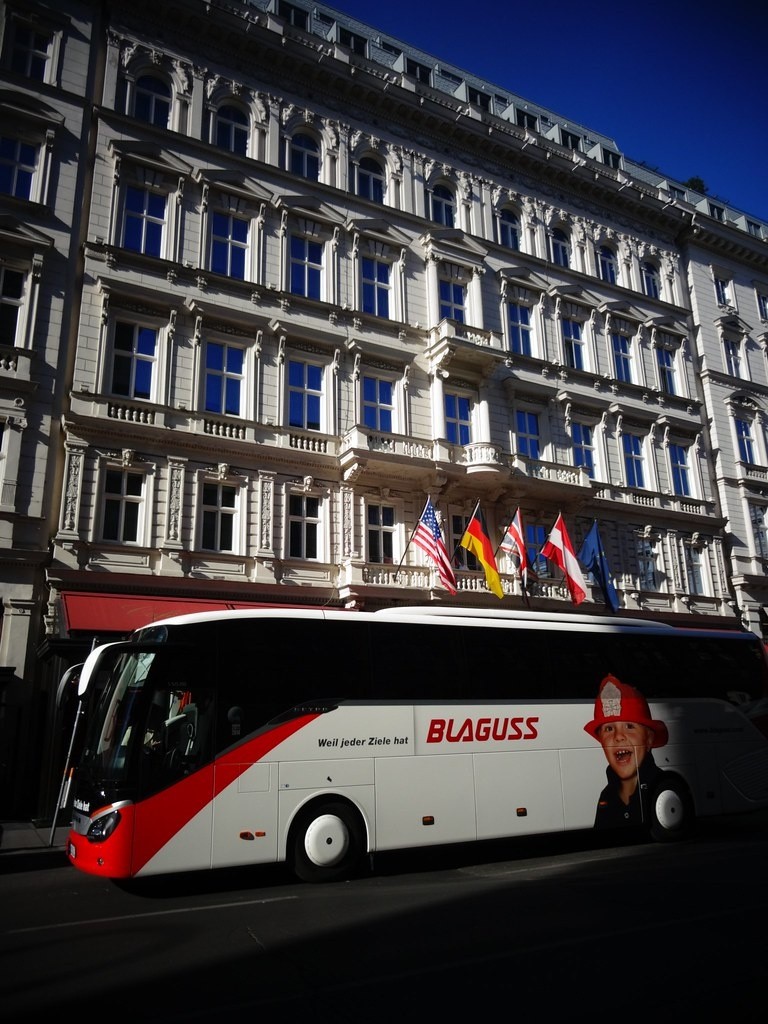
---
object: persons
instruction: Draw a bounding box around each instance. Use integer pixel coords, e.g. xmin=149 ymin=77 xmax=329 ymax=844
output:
xmin=583 ymin=672 xmax=697 ymax=828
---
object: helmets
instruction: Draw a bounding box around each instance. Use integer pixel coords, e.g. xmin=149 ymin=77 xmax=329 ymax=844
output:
xmin=584 ymin=676 xmax=669 ymax=750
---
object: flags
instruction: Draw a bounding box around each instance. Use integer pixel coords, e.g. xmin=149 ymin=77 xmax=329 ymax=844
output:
xmin=498 ymin=506 xmax=527 ymax=590
xmin=411 ymin=495 xmax=457 ymax=596
xmin=576 ymin=523 xmax=619 ymax=613
xmin=460 ymin=498 xmax=504 ymax=599
xmin=540 ymin=512 xmax=588 ymax=608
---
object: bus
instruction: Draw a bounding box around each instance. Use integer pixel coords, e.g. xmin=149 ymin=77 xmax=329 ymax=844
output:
xmin=56 ymin=606 xmax=768 ymax=884
